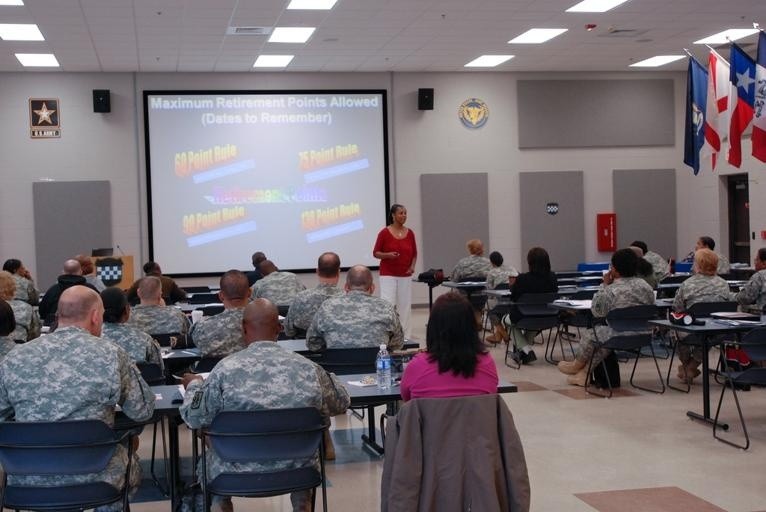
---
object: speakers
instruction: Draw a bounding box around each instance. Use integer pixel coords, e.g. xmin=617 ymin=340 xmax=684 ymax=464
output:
xmin=418 ymin=88 xmax=434 ymax=111
xmin=92 ymin=89 xmax=111 ymax=113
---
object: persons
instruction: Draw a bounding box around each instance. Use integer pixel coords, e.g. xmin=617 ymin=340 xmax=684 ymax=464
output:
xmin=703 ymin=47 xmax=733 ymax=173
xmin=373 ymin=204 xmax=418 ymax=341
xmin=448 ymin=239 xmax=492 ymax=332
xmin=397 ymin=231 xmax=403 ymax=236
xmin=725 ymin=44 xmax=756 ymax=169
xmin=629 ymin=240 xmax=670 ymax=278
xmin=683 ymin=56 xmax=708 ymax=176
xmin=734 ymin=248 xmax=766 ymax=314
xmin=485 ymin=251 xmax=519 ymax=344
xmin=191 ymin=251 xmax=401 ymax=458
xmin=508 ymin=246 xmax=559 ymax=364
xmin=179 ymin=297 xmax=350 ymax=512
xmin=751 ymin=32 xmax=766 ymax=163
xmin=399 ymin=292 xmax=498 ymax=401
xmin=556 ymin=249 xmax=654 ymax=388
xmin=626 ymin=246 xmax=658 ymax=288
xmin=671 ymin=249 xmax=730 ymax=383
xmin=1 ymin=254 xmax=190 ymax=448
xmin=0 ymin=285 xmax=156 ymax=512
xmin=691 ymin=237 xmax=729 ymax=274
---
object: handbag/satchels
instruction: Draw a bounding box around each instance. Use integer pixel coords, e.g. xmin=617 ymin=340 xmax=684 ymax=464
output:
xmin=594 ymin=358 xmax=621 ymax=388
xmin=417 ymin=267 xmax=444 ymax=280
xmin=725 ymin=344 xmax=752 ymax=391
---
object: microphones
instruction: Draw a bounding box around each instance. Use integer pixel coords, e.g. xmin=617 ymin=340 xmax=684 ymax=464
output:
xmin=117 ymin=245 xmax=124 ymax=256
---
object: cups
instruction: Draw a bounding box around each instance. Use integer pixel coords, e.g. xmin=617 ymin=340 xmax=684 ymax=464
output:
xmin=192 ymin=310 xmax=203 ymax=325
xmin=376 ymin=343 xmax=392 ymax=390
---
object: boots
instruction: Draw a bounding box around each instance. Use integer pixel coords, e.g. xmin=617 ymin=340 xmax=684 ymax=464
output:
xmin=485 ymin=328 xmax=503 ymax=344
xmin=566 ymin=369 xmax=591 ymax=387
xmin=324 ymin=429 xmax=336 ymax=460
xmin=678 ymin=360 xmax=701 ymax=376
xmin=558 ymin=359 xmax=586 ymax=375
xmin=474 ymin=310 xmax=483 ymax=330
xmin=496 ymin=323 xmax=511 ymax=346
xmin=677 ymin=358 xmax=701 ymax=379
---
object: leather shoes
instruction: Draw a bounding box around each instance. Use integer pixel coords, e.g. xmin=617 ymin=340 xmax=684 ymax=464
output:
xmin=507 ymin=351 xmax=521 ymax=363
xmin=522 ymin=351 xmax=538 ymax=364
